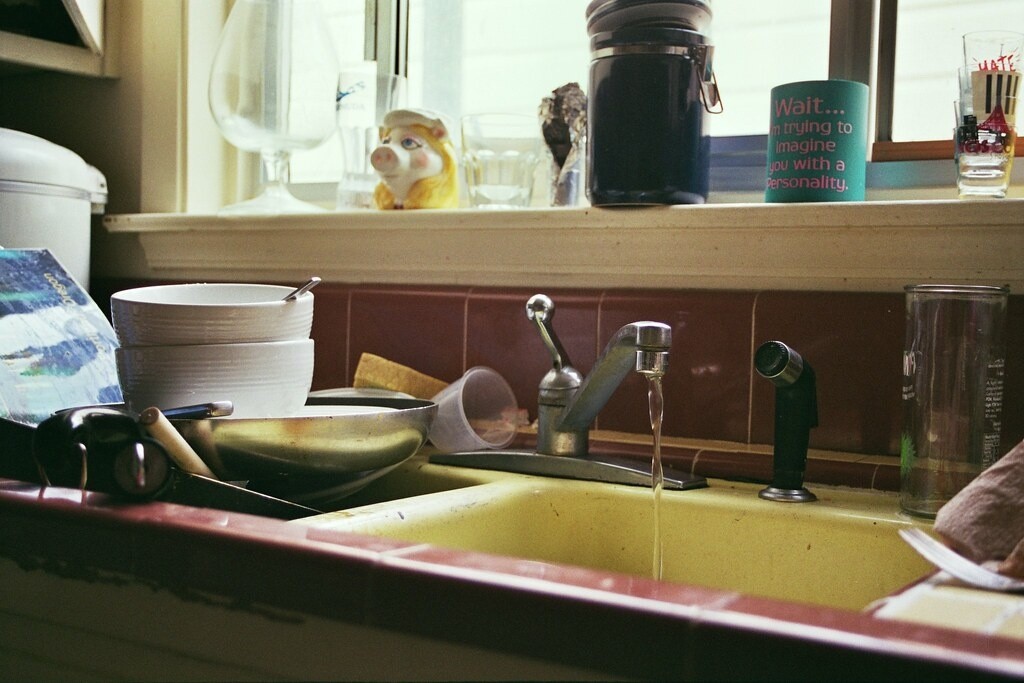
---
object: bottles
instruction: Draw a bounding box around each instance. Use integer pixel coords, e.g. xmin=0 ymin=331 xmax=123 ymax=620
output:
xmin=583 ymin=0 xmax=724 ymax=206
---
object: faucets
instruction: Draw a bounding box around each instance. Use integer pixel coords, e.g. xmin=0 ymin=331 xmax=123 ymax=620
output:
xmin=524 ymin=292 xmax=673 ymax=457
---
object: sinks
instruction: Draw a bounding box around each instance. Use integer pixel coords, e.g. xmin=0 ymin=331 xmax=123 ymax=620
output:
xmin=331 ymin=488 xmax=946 ymax=611
xmin=0 ymin=465 xmax=490 ymax=520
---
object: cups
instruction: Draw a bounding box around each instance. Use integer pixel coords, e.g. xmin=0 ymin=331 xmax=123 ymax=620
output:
xmin=457 ymin=113 xmax=549 ymax=210
xmin=901 ymin=278 xmax=1010 ymax=522
xmin=335 ymin=64 xmax=405 ymax=208
xmin=951 ymin=26 xmax=1024 ymax=199
xmin=424 ymin=366 xmax=516 ymax=453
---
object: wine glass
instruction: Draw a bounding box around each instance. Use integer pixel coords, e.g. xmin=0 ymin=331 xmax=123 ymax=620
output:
xmin=208 ymin=1 xmax=344 ymax=215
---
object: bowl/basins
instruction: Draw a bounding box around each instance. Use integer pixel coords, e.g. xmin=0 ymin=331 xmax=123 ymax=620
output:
xmin=111 ymin=283 xmax=313 ymax=421
xmin=150 ymin=395 xmax=438 ymax=504
